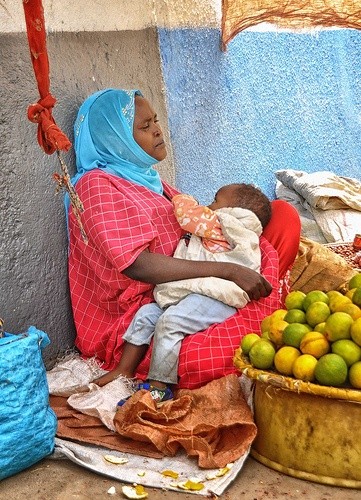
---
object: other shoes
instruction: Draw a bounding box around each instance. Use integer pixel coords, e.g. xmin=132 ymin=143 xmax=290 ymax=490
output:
xmin=116 ymin=382 xmax=174 ymax=411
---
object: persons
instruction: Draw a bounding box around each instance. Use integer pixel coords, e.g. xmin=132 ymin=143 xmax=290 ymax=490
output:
xmin=61 ymin=85 xmax=307 ymax=391
xmin=77 ymin=179 xmax=273 ymax=415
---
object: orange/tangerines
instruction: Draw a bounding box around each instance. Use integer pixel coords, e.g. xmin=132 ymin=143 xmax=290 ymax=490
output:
xmin=240 ymin=274 xmax=361 ymax=388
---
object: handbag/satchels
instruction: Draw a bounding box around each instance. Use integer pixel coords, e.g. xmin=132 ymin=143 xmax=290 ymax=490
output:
xmin=1 ymin=323 xmax=59 ymax=481
